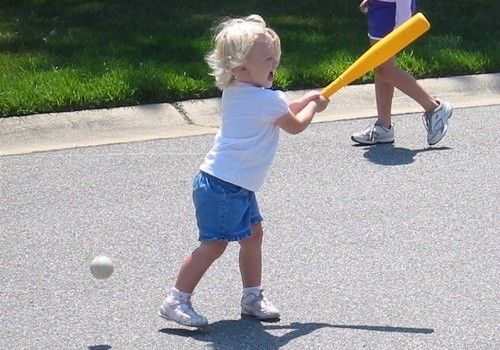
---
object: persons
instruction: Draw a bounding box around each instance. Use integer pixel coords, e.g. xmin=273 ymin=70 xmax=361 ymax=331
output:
xmin=351 ymin=0 xmax=454 ymax=145
xmin=156 ymin=14 xmax=330 ymax=328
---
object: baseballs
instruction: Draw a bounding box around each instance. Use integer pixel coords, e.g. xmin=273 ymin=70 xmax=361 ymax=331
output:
xmin=89 ymin=255 xmax=114 ymax=280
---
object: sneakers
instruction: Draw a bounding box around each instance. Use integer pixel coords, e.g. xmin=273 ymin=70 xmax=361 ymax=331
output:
xmin=421 ymin=96 xmax=453 ymax=145
xmin=351 ymin=124 xmax=395 ymax=145
xmin=240 ymin=288 xmax=280 ymax=319
xmin=158 ymin=297 xmax=208 ymax=328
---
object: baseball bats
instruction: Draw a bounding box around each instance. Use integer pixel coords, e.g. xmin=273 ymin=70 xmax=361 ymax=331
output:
xmin=318 ymin=12 xmax=432 ymax=99
